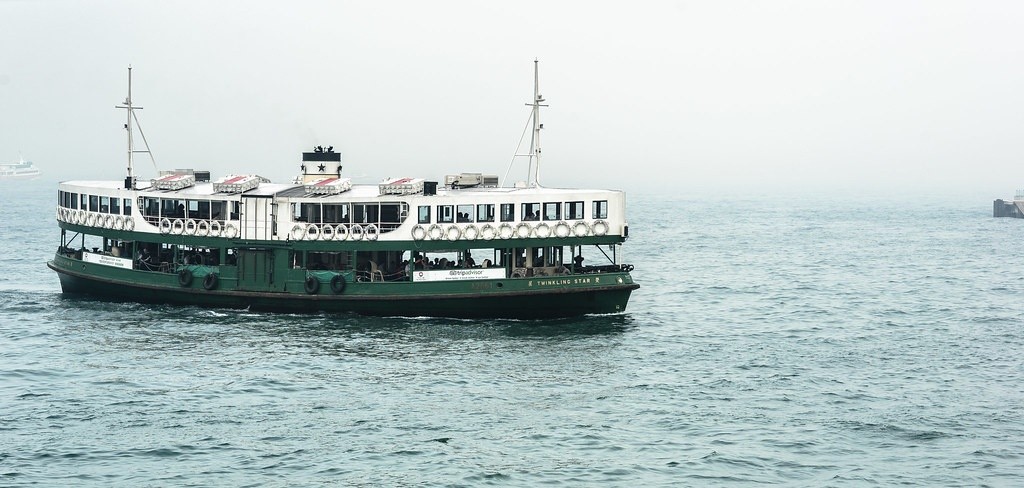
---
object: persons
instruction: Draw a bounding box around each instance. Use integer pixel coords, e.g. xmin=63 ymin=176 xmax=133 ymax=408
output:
xmin=138 ymin=248 xmax=151 ymax=270
xmin=457 ymin=212 xmax=469 ymax=222
xmin=377 ymin=253 xmax=488 ymax=281
xmin=523 ymin=210 xmax=550 ymax=221
xmin=344 ymin=214 xmax=349 ymax=223
xmin=92 ymin=200 xmax=98 ymax=211
xmin=177 ymin=204 xmax=185 ymax=219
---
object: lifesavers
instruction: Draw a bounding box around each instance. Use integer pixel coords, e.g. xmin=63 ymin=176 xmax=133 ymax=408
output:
xmin=510 ymin=270 xmax=524 ymax=278
xmin=56 ymin=207 xmax=134 ymax=232
xmin=412 ymin=219 xmax=609 ymax=242
xmin=178 ymin=269 xmax=192 ymax=286
xmin=203 ymin=273 xmax=217 ymax=290
xmin=304 ymin=276 xmax=320 ymax=294
xmin=225 ymin=223 xmax=237 ymax=239
xmin=159 ymin=218 xmax=222 ymax=237
xmin=330 ymin=275 xmax=346 ymax=293
xmin=291 ymin=224 xmax=379 ymax=242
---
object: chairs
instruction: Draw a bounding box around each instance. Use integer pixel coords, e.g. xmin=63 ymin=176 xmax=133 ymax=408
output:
xmin=82 ymin=204 xmax=131 ymax=215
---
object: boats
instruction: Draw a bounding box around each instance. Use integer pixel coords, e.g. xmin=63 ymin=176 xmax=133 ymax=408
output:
xmin=45 ymin=56 xmax=641 ymax=320
xmin=0 ymin=153 xmax=43 ymax=176
